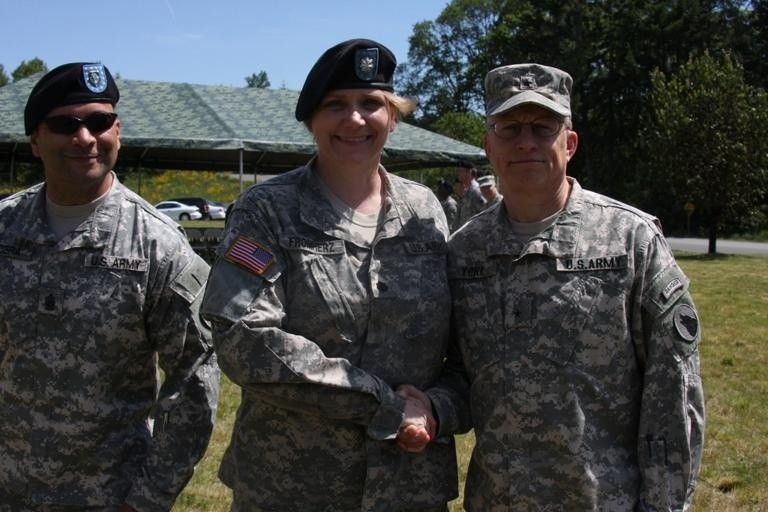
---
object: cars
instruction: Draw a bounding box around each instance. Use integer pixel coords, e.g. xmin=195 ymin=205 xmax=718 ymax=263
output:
xmin=155 ymin=197 xmax=227 ymax=222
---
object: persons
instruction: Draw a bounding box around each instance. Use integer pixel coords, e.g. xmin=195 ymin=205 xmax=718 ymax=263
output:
xmin=455 ymin=161 xmax=486 ymax=227
xmin=1 ymin=60 xmax=221 ymax=512
xmin=473 ymin=175 xmax=504 ymax=209
xmin=197 ymin=36 xmax=454 ymax=511
xmin=453 ymin=178 xmax=469 ymax=204
xmin=395 ymin=61 xmax=707 ymax=512
xmin=434 ymin=175 xmax=457 ymax=230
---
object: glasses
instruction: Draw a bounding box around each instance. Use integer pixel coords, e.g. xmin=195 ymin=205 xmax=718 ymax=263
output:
xmin=39 ymin=111 xmax=118 ymax=135
xmin=485 ymin=113 xmax=565 ymax=142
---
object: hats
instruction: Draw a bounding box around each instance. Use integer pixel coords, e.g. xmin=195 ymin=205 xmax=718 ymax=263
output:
xmin=294 ymin=39 xmax=397 ymax=122
xmin=484 ymin=63 xmax=574 ymax=121
xmin=24 ymin=63 xmax=121 ymax=136
xmin=437 ymin=161 xmax=497 ymax=194
